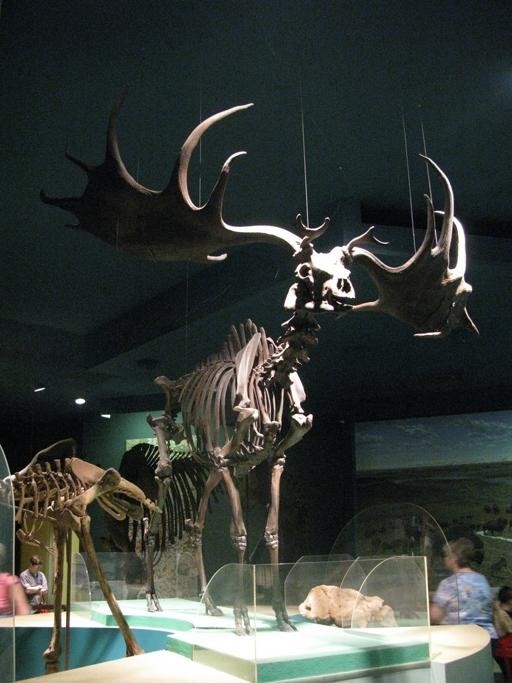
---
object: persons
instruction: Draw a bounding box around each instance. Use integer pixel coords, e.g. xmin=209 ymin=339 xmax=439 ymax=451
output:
xmin=427 ymin=534 xmax=497 ymax=651
xmin=1 ymin=542 xmax=30 ymax=616
xmin=18 ymin=555 xmax=48 ymax=615
xmin=494 ymin=585 xmax=512 ymax=659
